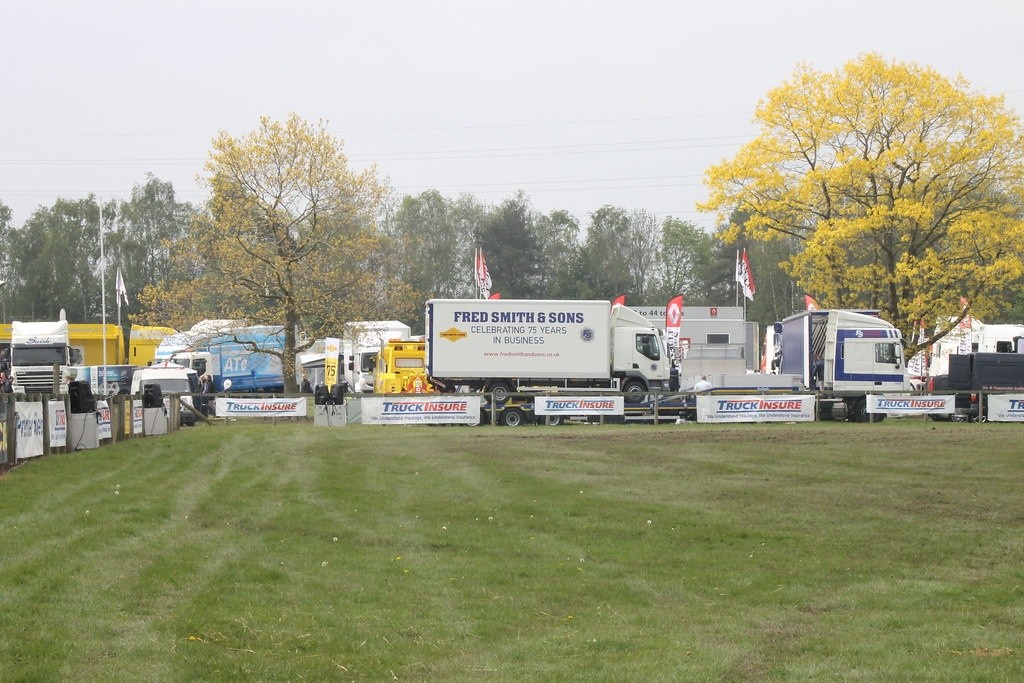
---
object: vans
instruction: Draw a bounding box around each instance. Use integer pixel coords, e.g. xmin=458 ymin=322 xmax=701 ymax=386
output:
xmin=949 ymin=353 xmax=1024 ymax=422
xmin=131 ymin=370 xmax=194 ymax=427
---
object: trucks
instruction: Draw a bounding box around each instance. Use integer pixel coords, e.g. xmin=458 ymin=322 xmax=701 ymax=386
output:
xmin=173 ymin=324 xmax=301 ymax=392
xmin=14 ymin=320 xmax=67 ymax=398
xmin=424 ymin=297 xmax=671 ymax=402
xmin=355 ymin=331 xmax=411 ymax=394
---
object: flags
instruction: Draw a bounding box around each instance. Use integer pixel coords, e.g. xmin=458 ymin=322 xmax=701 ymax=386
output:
xmin=473 ymin=246 xmax=494 ymax=299
xmin=116 ymin=267 xmax=130 ymax=307
xmin=735 ymin=250 xmax=756 ymax=304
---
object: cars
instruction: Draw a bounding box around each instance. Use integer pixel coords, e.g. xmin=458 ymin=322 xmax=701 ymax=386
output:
xmin=924 ymin=375 xmax=964 ymax=421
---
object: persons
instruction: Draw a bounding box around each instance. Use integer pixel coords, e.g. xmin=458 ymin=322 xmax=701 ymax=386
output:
xmin=200 ymin=375 xmax=220 ymax=418
xmin=669 ymin=364 xmax=679 ymax=392
xmin=301 ymin=374 xmax=313 ymax=393
xmin=694 ymin=375 xmax=712 ymax=392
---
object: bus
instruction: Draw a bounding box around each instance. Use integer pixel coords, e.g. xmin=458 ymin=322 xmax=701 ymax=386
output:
xmin=370 ymin=339 xmax=433 ymax=393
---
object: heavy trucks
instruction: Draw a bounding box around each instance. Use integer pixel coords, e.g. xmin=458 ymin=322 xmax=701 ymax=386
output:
xmin=426 ymin=310 xmax=909 ymax=421
